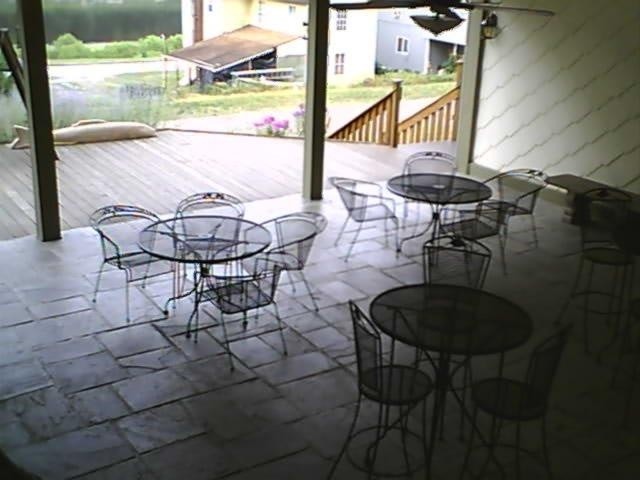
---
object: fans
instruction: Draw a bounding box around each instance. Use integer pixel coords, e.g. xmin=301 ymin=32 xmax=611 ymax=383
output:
xmin=327 ymin=0 xmax=557 ymax=17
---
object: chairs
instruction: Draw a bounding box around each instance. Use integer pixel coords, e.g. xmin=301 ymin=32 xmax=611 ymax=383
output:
xmin=242 ymin=210 xmax=329 ymax=318
xmin=170 ymin=190 xmax=247 ymax=309
xmin=186 ymin=256 xmax=289 ymax=373
xmin=89 ymin=200 xmax=177 ymax=324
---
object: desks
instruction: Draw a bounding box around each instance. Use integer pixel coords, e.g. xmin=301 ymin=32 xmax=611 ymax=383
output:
xmin=135 ymin=214 xmax=273 ymax=338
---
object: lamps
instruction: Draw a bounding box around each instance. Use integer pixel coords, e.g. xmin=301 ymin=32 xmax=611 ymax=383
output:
xmin=479 ymin=12 xmax=498 ymax=38
xmin=408 ymin=11 xmax=467 ymax=38
